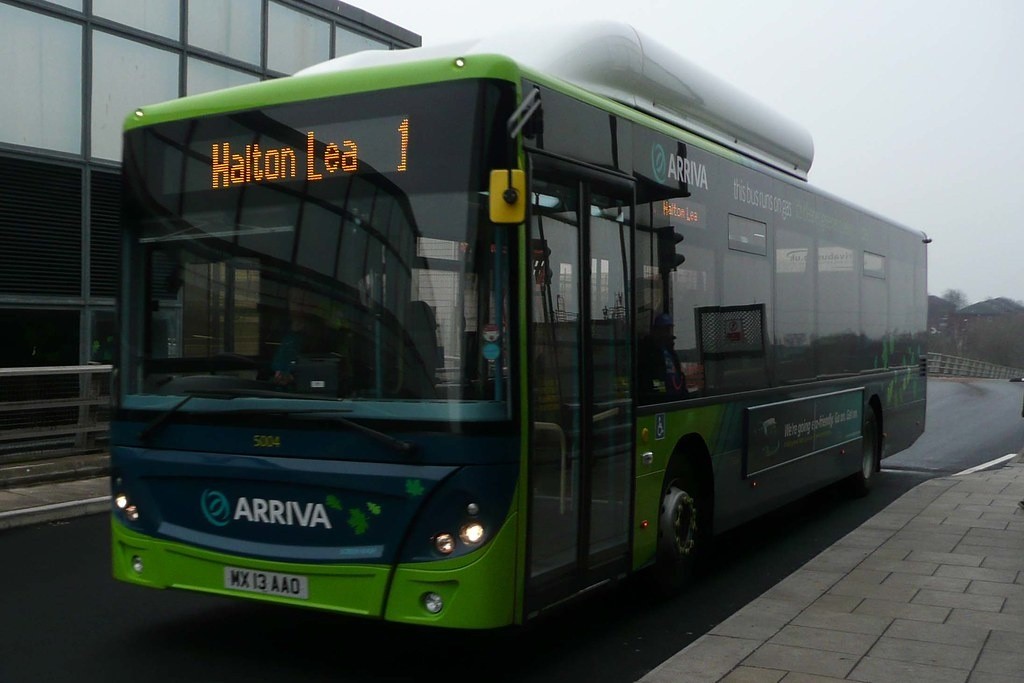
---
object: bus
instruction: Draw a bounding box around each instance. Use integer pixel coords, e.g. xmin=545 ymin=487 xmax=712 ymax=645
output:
xmin=110 ymin=24 xmax=932 ymax=628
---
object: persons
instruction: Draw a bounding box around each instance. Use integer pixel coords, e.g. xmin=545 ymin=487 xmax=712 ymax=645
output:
xmin=639 ymin=314 xmax=689 ymax=401
xmin=260 ymin=282 xmax=348 ymax=392
